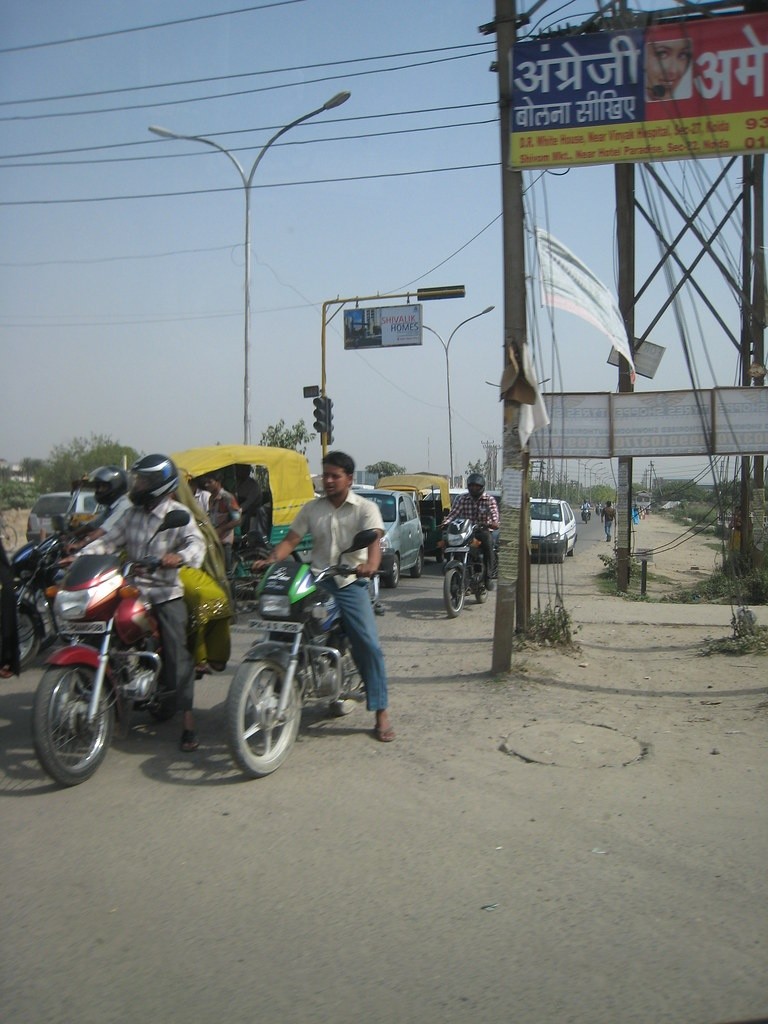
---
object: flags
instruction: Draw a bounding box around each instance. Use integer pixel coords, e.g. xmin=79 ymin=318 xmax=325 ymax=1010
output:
xmin=537 ymin=228 xmax=636 ymax=384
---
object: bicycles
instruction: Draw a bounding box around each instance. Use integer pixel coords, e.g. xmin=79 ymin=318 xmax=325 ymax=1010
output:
xmin=0 ymin=515 xmax=17 ymax=552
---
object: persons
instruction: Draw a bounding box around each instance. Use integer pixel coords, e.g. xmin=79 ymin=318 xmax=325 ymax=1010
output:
xmin=643 ymin=39 xmax=692 ymax=102
xmin=596 ymin=502 xmax=602 ymax=509
xmin=632 ymin=505 xmax=646 ymax=526
xmin=40 ymin=516 xmax=64 ymax=542
xmin=58 ymin=453 xmax=207 ymax=752
xmin=580 ymin=499 xmax=591 ymax=521
xmin=62 ymin=465 xmax=129 ymax=554
xmin=601 ymin=501 xmax=617 ymax=542
xmin=435 ymin=474 xmax=499 ymax=591
xmin=188 ymin=464 xmax=263 ymax=625
xmin=251 ymin=451 xmax=396 ymax=742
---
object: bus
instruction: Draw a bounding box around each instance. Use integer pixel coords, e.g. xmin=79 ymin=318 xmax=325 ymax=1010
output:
xmin=634 ymin=493 xmax=650 ymax=515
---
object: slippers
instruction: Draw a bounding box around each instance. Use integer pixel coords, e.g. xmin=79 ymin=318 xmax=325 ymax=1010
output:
xmin=374 ymin=721 xmax=396 ymax=741
xmin=180 ymin=729 xmax=201 ymax=752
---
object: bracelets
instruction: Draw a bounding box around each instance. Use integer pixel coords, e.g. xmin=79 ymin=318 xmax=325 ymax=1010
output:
xmin=83 ymin=536 xmax=93 ymax=543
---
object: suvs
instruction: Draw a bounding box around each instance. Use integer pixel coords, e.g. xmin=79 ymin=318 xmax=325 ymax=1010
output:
xmin=528 ymin=498 xmax=576 ymax=562
xmin=348 ymin=488 xmax=423 ymax=588
xmin=26 ymin=492 xmax=98 ymax=544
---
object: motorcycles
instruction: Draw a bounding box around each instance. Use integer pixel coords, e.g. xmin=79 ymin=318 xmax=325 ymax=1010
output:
xmin=227 ymin=525 xmax=383 ymax=777
xmin=12 ymin=486 xmax=97 ymax=668
xmin=596 ymin=505 xmax=602 ymax=516
xmin=436 ymin=519 xmax=498 ymax=620
xmin=374 ymin=476 xmax=450 ymax=562
xmin=32 ymin=510 xmax=181 ymax=790
xmin=171 ymin=447 xmax=313 ymax=612
xmin=580 ymin=505 xmax=593 ymax=524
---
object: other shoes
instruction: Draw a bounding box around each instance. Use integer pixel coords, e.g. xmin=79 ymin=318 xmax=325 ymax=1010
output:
xmin=606 ymin=535 xmax=612 ymax=542
xmin=484 ymin=576 xmax=495 ymax=591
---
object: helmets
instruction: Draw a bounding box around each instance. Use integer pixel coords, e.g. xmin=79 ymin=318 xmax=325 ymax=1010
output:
xmin=129 ymin=454 xmax=179 ymax=506
xmin=466 ymin=474 xmax=486 ymax=498
xmin=88 ymin=465 xmax=128 ymax=505
xmin=584 ymin=499 xmax=588 ymax=503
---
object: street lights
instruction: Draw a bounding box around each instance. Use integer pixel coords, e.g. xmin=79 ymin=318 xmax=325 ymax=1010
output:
xmin=147 ymin=90 xmax=351 ymax=450
xmin=422 ymin=305 xmax=495 ymax=489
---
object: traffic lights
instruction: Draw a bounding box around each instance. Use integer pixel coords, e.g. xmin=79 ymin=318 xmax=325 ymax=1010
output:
xmin=313 ymin=398 xmax=334 ymax=445
